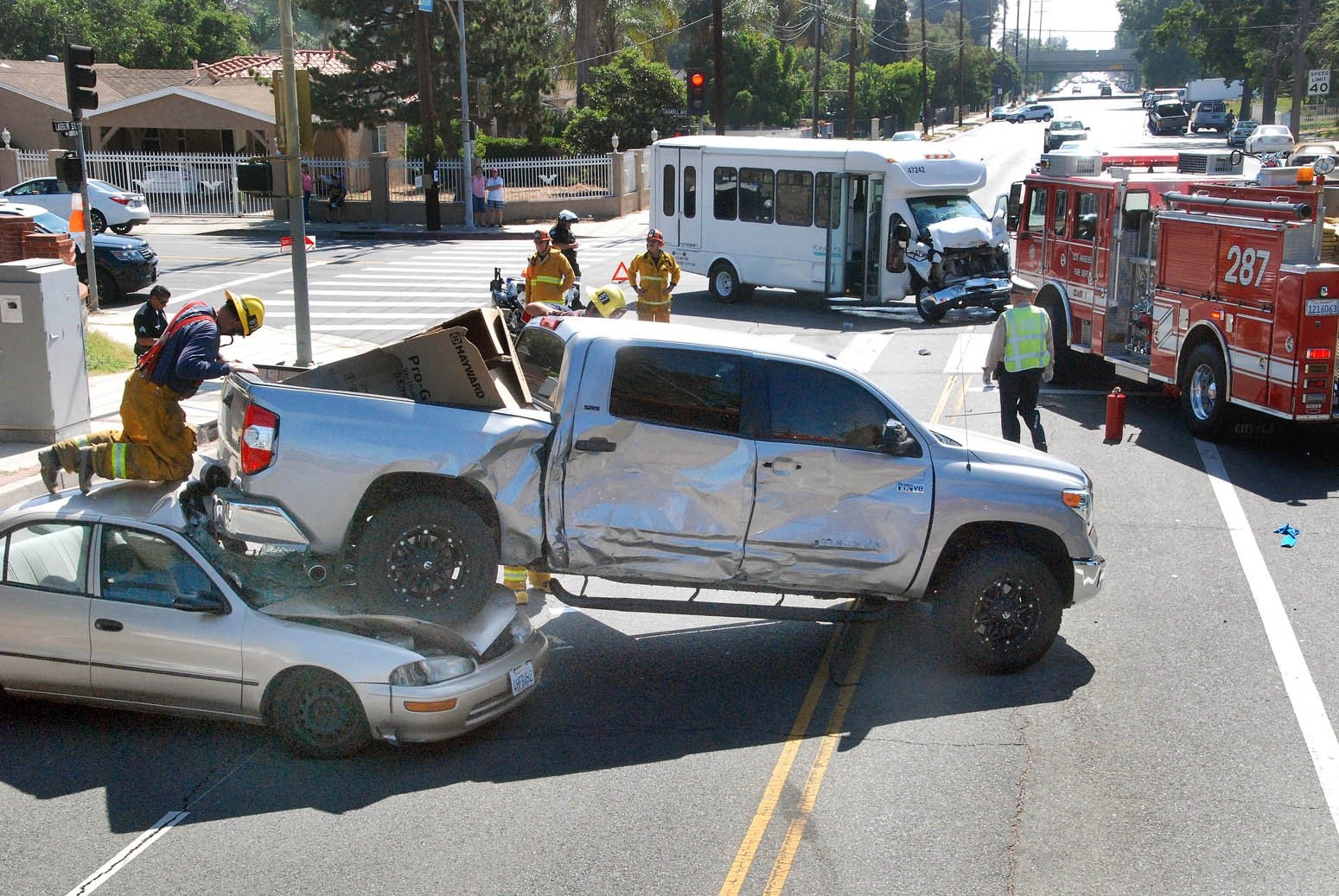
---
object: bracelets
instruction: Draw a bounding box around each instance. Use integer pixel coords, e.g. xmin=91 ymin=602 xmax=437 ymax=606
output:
xmin=1045 ymin=368 xmax=1053 ymax=373
xmin=668 ymin=287 xmax=672 ymax=291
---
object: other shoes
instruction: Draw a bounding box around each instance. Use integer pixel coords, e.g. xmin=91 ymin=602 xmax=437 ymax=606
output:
xmin=335 ymin=220 xmax=342 ymax=224
xmin=324 ymin=219 xmax=332 ymax=223
xmin=499 ymin=223 xmax=502 ymax=228
xmin=1036 ymin=442 xmax=1047 ymax=452
xmin=487 ymin=222 xmax=494 ymax=229
xmin=481 ymin=221 xmax=486 ymax=226
xmin=305 ymin=220 xmax=312 ymax=224
xmin=473 ymin=222 xmax=477 ymax=227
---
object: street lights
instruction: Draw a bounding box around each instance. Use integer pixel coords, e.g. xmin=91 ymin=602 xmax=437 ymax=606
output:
xmin=958 ymin=15 xmax=991 ymax=126
xmin=922 ymin=0 xmax=959 ymax=137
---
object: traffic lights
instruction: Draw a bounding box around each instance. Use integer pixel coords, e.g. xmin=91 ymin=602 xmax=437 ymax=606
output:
xmin=55 ymin=156 xmax=83 ymax=185
xmin=63 ymin=34 xmax=99 ymax=114
xmin=686 ymin=68 xmax=707 ymax=116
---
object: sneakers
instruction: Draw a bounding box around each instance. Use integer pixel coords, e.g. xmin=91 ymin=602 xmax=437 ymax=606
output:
xmin=78 ymin=446 xmax=94 ymax=493
xmin=38 ymin=448 xmax=61 ymax=494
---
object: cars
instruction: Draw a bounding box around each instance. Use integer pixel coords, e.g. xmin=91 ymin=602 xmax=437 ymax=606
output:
xmin=1043 ymin=119 xmax=1091 ymax=154
xmin=1283 ymin=141 xmax=1339 ymax=170
xmin=1026 ymin=95 xmax=1038 ymax=105
xmin=891 ymin=131 xmax=924 ymax=144
xmin=1072 ymin=86 xmax=1080 ymax=93
xmin=1224 ymin=120 xmax=1263 ymax=147
xmin=1 ymin=203 xmax=159 ymax=305
xmin=1049 ymin=76 xmax=1135 ymax=96
xmin=0 ymin=177 xmax=151 ymax=234
xmin=991 ymin=107 xmax=1008 ymax=122
xmin=1241 ymin=124 xmax=1294 ymax=155
xmin=1 ymin=481 xmax=553 ymax=761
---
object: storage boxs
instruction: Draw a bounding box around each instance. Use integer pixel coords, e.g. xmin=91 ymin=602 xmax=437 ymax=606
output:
xmin=277 ymin=305 xmax=532 ymax=408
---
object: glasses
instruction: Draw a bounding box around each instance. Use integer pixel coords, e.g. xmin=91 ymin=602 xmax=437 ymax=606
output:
xmin=157 ymin=296 xmax=166 ymax=306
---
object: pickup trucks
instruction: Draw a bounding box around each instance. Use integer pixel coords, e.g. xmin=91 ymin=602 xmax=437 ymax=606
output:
xmin=1190 ymin=101 xmax=1226 ymax=133
xmin=212 ymin=313 xmax=1105 ymax=676
xmin=1148 ymin=100 xmax=1189 ymax=135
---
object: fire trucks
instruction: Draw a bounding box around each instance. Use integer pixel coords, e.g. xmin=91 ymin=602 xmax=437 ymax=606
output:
xmin=1004 ymin=140 xmax=1339 ymax=441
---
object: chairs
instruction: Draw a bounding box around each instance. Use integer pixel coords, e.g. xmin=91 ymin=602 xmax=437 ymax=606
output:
xmin=1054 ymin=124 xmax=1060 ymax=127
xmin=1253 ymin=125 xmax=1257 ymax=127
xmin=1072 ymin=123 xmax=1078 ymax=127
xmin=103 ymin=543 xmax=145 ymax=600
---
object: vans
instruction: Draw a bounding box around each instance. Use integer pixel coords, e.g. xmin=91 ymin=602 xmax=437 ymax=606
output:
xmin=1142 ymin=86 xmax=1187 ymax=108
xmin=648 ymin=135 xmax=1014 ymax=324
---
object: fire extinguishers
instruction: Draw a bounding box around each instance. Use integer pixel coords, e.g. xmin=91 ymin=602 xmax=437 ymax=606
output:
xmin=1105 ymin=387 xmax=1126 ymax=440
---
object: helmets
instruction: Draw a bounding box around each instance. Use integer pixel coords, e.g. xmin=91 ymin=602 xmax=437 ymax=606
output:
xmin=585 ymin=285 xmax=628 ymax=319
xmin=225 ymin=289 xmax=265 ymax=338
xmin=533 ymin=230 xmax=554 ymax=242
xmin=646 ymin=229 xmax=665 ymax=247
xmin=558 ymin=210 xmax=579 ymax=223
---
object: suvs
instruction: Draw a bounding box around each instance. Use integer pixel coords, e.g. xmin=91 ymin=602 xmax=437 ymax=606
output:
xmin=1100 ymin=86 xmax=1112 ymax=98
xmin=1006 ymin=104 xmax=1055 ymax=124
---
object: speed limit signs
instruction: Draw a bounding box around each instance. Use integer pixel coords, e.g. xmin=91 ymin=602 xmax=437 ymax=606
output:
xmin=1308 ymin=70 xmax=1330 ymax=97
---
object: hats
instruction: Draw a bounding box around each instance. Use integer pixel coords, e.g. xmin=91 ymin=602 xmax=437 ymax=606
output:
xmin=1010 ymin=276 xmax=1040 ymax=295
xmin=491 ymin=168 xmax=497 ymax=172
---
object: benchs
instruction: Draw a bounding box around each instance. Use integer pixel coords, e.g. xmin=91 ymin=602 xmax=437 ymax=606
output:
xmin=0 ymin=525 xmax=144 ymax=592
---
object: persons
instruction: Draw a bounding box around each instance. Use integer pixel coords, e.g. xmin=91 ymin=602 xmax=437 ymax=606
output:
xmin=523 ymin=283 xmax=629 ymax=319
xmin=38 ymin=289 xmax=265 ymax=492
xmin=981 ymin=273 xmax=1053 ymax=454
xmin=549 ymin=209 xmax=587 ymax=311
xmin=325 ymin=176 xmax=345 ymax=224
xmin=472 ymin=166 xmax=504 ymax=228
xmin=133 ymin=285 xmax=172 ymax=367
xmin=523 ymin=230 xmax=576 ymax=316
xmin=1224 ymin=108 xmax=1235 ymax=139
xmin=627 ymin=229 xmax=680 ymax=324
xmin=300 ymin=163 xmax=317 ymax=223
